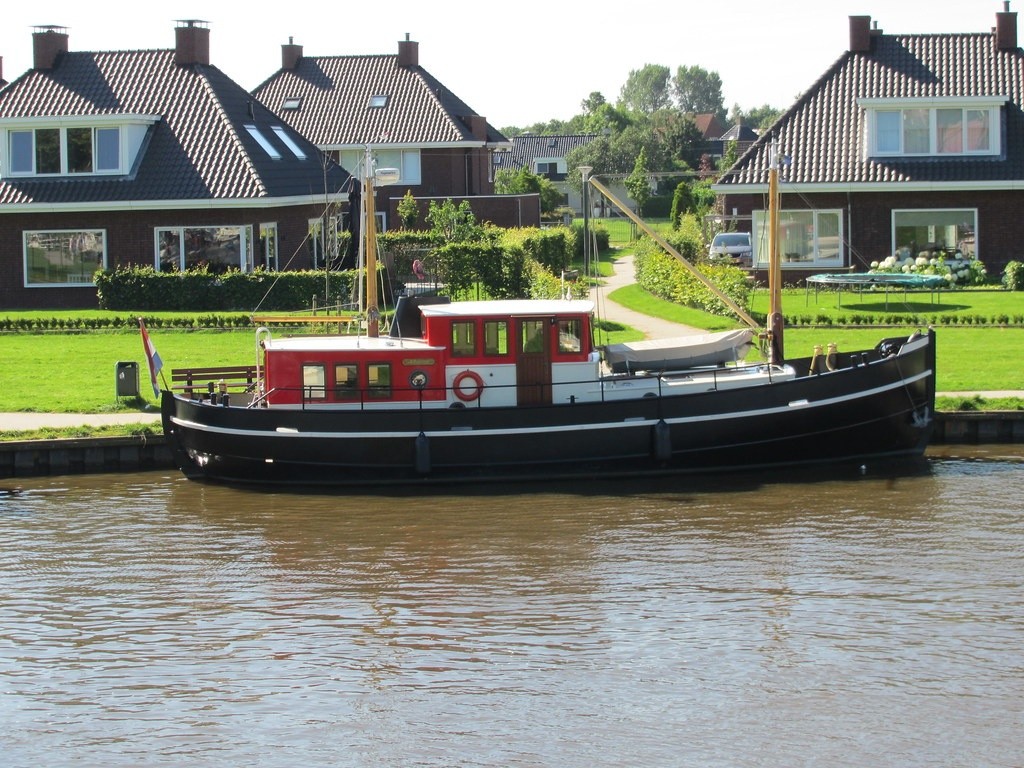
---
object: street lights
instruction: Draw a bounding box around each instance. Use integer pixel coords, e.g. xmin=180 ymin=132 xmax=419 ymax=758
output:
xmin=575 ymin=165 xmax=594 ymax=276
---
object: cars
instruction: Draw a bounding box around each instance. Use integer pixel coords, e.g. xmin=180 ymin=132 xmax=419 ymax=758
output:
xmin=705 ymin=232 xmax=752 ymax=266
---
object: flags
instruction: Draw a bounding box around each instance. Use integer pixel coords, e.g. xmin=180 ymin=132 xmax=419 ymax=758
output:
xmin=141 ymin=323 xmax=165 ymax=400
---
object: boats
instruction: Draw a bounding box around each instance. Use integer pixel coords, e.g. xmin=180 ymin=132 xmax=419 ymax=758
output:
xmin=159 ymin=140 xmax=939 ymax=496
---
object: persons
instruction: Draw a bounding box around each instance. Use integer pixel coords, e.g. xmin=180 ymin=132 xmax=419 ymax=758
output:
xmin=260 ymin=235 xmax=266 ymax=255
xmin=413 ymin=260 xmax=429 ymax=280
xmin=522 ymin=328 xmax=544 ymax=355
xmin=309 ymin=230 xmax=334 ymax=252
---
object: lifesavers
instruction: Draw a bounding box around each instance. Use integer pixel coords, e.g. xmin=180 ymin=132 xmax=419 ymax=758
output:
xmin=453 ymin=371 xmax=483 ymax=401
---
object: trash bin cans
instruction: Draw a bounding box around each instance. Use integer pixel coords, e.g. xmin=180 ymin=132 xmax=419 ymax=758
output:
xmin=115 ymin=362 xmax=140 ymax=402
xmin=404 ymin=283 xmax=444 ymax=298
